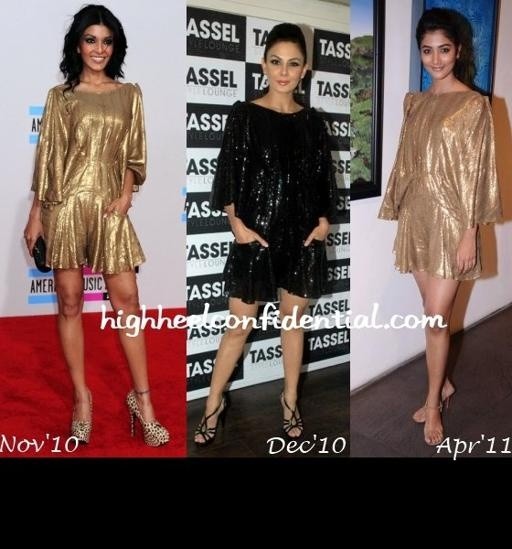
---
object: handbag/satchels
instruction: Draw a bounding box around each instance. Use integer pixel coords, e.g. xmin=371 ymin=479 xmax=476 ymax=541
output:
xmin=31 ymin=235 xmax=52 ymax=273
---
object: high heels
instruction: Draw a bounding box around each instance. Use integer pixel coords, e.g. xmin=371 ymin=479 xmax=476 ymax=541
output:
xmin=278 ymin=388 xmax=305 ymax=442
xmin=192 ymin=394 xmax=228 ymax=447
xmin=411 ymin=382 xmax=456 ymax=424
xmin=125 ymin=388 xmax=170 ymax=448
xmin=70 ymin=384 xmax=93 ymax=444
xmin=422 ymin=398 xmax=445 ymax=446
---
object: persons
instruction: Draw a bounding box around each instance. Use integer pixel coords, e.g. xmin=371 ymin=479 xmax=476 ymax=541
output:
xmin=376 ymin=6 xmax=502 ymax=447
xmin=23 ymin=6 xmax=170 ymax=448
xmin=193 ymin=22 xmax=335 ymax=447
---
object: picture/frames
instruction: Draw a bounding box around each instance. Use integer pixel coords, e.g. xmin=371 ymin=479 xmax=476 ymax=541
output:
xmin=351 ymin=0 xmax=387 ymax=203
xmin=420 ymin=0 xmax=501 ymax=106
